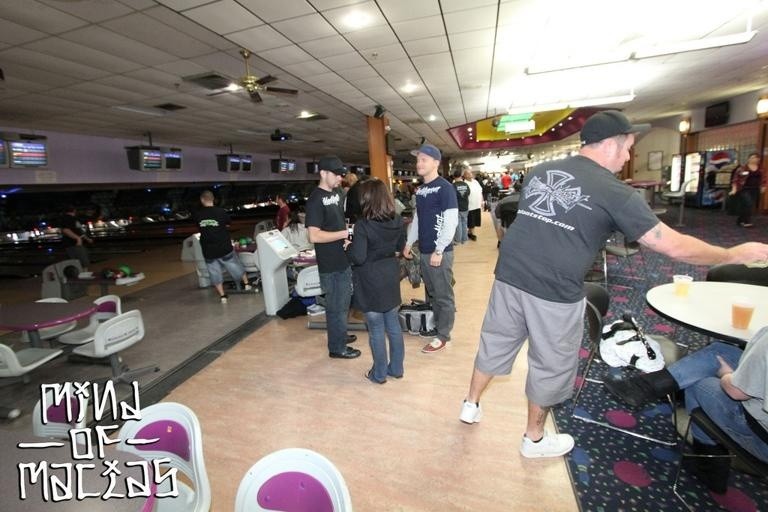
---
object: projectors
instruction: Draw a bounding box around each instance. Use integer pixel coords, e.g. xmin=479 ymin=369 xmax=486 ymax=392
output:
xmin=271 ymin=128 xmax=292 ymax=141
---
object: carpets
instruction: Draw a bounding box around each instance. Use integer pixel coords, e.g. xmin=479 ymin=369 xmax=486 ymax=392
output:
xmin=488 ymin=201 xmax=768 ymax=512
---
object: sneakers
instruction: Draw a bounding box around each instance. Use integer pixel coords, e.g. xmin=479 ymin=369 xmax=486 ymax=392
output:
xmin=220 ymin=297 xmax=228 ymax=304
xmin=395 ymin=375 xmax=404 ymax=378
xmin=245 ymin=285 xmax=252 ymax=291
xmin=520 ymin=429 xmax=574 ymax=458
xmin=459 ymin=399 xmax=482 ymax=423
xmin=307 ymin=304 xmax=326 ymax=317
xmin=739 ymin=222 xmax=753 ymax=227
xmin=365 ymin=369 xmax=386 ymax=384
xmin=421 ymin=338 xmax=446 ymax=353
xmin=420 ymin=328 xmax=438 ymax=336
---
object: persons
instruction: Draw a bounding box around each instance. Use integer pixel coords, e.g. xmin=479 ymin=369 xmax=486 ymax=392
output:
xmin=273 ymin=165 xmax=530 ymax=315
xmin=343 ymin=179 xmax=408 ymax=383
xmin=460 ymin=110 xmax=768 ymax=458
xmin=194 ymin=190 xmax=259 ymax=304
xmin=62 ymin=203 xmax=93 ymax=267
xmin=602 ymin=326 xmax=768 ymax=495
xmin=729 ymin=153 xmax=767 ymax=229
xmin=402 ymin=145 xmax=460 ymax=354
xmin=303 ymin=154 xmax=361 ymax=360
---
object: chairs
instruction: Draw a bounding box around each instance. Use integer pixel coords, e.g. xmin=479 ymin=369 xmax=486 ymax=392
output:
xmin=181 ymin=220 xmax=367 ymax=330
xmin=706 ymin=264 xmax=768 ymax=348
xmin=20 ymin=298 xmax=77 ymax=349
xmin=0 ymin=344 xmax=63 ymax=421
xmin=58 ymin=295 xmax=122 ymax=364
xmin=72 ymin=309 xmax=160 ymax=405
xmin=570 ymin=283 xmax=688 ymax=447
xmin=115 ymin=402 xmax=212 ymax=512
xmin=601 ymin=178 xmax=695 ymax=289
xmin=32 ymin=385 xmax=88 ymax=445
xmin=234 ymin=448 xmax=353 ymax=512
xmin=673 ymin=406 xmax=768 ymax=512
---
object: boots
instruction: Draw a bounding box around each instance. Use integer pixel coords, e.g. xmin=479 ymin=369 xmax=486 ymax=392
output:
xmin=683 ymin=437 xmax=730 ymax=495
xmin=600 ymin=369 xmax=679 ymax=409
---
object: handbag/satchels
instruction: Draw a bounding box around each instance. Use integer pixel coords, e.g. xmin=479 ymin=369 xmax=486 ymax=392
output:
xmin=398 ymin=298 xmax=435 ymax=332
xmin=599 ymin=314 xmax=688 ymax=374
xmin=278 ymin=289 xmax=315 ymax=319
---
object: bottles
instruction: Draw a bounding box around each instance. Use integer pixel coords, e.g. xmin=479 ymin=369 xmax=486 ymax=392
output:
xmin=239 ymin=235 xmax=247 ymax=248
xmin=348 ymin=225 xmax=353 ymax=242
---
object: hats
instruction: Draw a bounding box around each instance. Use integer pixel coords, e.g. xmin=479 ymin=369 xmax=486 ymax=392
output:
xmin=318 ymin=155 xmax=348 ymax=174
xmin=454 ymin=171 xmax=461 ymax=178
xmin=409 ymin=143 xmax=442 ymax=161
xmin=580 ymin=110 xmax=652 ymax=144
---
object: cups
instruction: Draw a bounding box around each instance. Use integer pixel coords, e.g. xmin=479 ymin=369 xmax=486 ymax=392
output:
xmin=731 ymin=298 xmax=755 ymax=330
xmin=673 ymin=275 xmax=694 ymax=297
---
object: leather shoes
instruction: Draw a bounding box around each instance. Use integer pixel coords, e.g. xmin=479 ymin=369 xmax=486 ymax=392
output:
xmin=330 ymin=346 xmax=361 ymax=358
xmin=468 ymin=230 xmax=477 ymax=240
xmin=346 ymin=335 xmax=357 ymax=344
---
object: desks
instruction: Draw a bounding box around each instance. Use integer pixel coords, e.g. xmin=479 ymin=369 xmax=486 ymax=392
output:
xmin=0 ymin=431 xmax=152 ymax=512
xmin=0 ymin=300 xmax=98 ymax=347
xmin=646 ymin=281 xmax=768 ymax=444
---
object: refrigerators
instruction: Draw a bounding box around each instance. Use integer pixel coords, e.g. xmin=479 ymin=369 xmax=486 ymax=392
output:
xmin=682 ymin=148 xmax=740 ymax=209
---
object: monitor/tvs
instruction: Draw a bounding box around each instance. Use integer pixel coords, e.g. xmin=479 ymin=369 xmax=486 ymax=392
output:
xmin=307 ymin=162 xmax=318 ymax=174
xmin=271 ymin=159 xmax=297 ymax=176
xmin=123 ymin=146 xmax=183 ymax=172
xmin=217 ymin=154 xmax=254 ymax=174
xmin=705 ymin=100 xmax=729 ymax=126
xmin=0 ymin=131 xmax=50 ymax=169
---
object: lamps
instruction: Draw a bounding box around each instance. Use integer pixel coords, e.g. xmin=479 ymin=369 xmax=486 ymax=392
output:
xmin=524 ymin=53 xmax=631 ymax=74
xmin=633 ymin=30 xmax=758 ymax=59
xmin=568 ymin=95 xmax=636 ymax=108
xmin=507 ymin=101 xmax=569 ymax=113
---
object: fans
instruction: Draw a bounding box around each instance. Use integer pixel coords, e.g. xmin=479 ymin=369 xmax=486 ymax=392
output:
xmin=205 ymin=50 xmax=298 ymax=103
